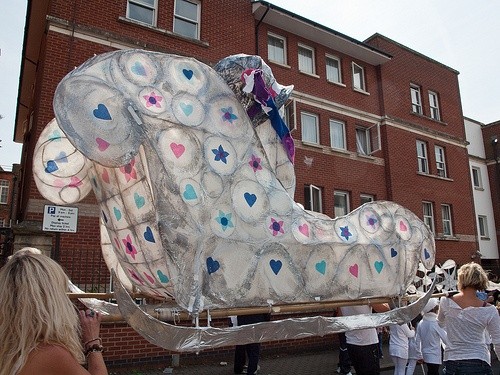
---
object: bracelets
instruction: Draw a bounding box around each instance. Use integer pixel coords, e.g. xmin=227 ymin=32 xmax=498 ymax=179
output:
xmin=84 ymin=338 xmax=103 ymax=357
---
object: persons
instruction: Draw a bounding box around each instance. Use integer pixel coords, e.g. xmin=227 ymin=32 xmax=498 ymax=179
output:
xmin=0 ymin=247 xmax=108 ymax=375
xmin=333 ymin=262 xmax=500 ymax=375
xmin=228 ymin=313 xmax=270 ymax=375
xmin=436 ymin=263 xmax=500 ymax=375
